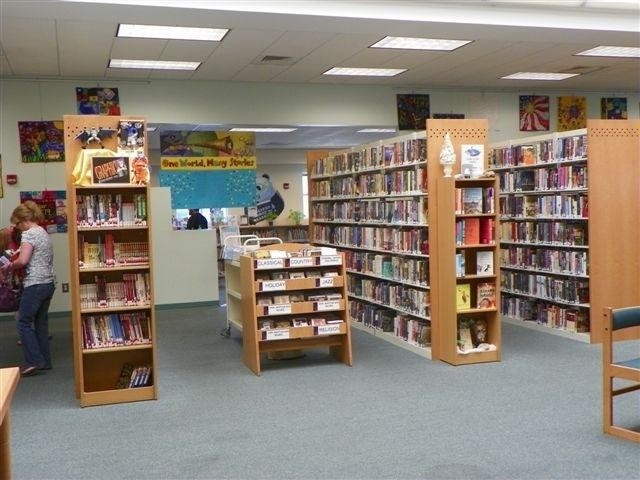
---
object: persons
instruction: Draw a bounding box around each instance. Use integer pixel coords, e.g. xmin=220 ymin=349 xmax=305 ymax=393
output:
xmin=0 ymin=201 xmax=55 ymax=377
xmin=187 ymin=208 xmax=208 ymax=229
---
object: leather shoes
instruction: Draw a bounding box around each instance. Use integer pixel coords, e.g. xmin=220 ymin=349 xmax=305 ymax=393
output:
xmin=21 ymin=357 xmax=52 ymax=378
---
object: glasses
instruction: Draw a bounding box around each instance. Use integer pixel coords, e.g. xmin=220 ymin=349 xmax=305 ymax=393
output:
xmin=15 ymin=222 xmax=20 ymax=226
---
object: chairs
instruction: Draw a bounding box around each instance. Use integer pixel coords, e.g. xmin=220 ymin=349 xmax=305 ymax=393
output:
xmin=600 ymin=304 xmax=640 ymax=442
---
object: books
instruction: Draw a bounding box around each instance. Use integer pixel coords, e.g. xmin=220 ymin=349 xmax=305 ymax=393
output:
xmin=312 ymin=138 xmax=431 ymax=349
xmin=253 ymin=248 xmax=344 ymax=344
xmin=77 ymin=194 xmax=153 ymax=391
xmin=488 ymin=136 xmax=590 ymax=335
xmin=453 ymin=186 xmax=497 ymax=351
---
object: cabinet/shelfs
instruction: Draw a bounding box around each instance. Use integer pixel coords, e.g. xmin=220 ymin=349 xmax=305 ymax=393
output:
xmin=439 ymin=170 xmax=504 ymax=366
xmin=61 ymin=115 xmax=158 ymax=410
xmin=238 ymin=244 xmax=356 ymax=369
xmin=303 ymin=118 xmax=493 ymax=362
xmin=483 ymin=117 xmax=640 ymax=345
